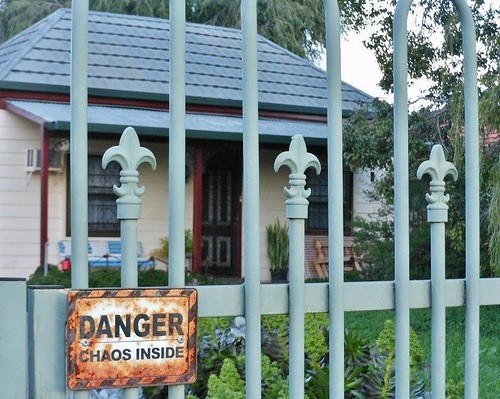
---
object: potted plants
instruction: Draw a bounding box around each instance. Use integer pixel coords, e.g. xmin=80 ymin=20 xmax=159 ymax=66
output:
xmin=265 ymin=215 xmax=289 ymax=282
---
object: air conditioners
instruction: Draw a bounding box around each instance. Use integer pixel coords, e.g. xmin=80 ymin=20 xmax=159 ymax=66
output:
xmin=26 ymin=148 xmax=64 ymax=174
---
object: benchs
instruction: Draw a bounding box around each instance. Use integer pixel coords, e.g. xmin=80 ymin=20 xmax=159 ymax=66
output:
xmin=57 ymin=236 xmax=157 ymax=272
